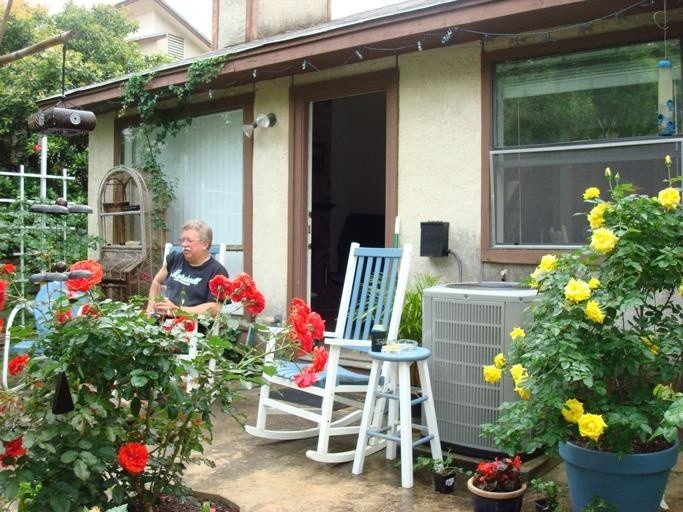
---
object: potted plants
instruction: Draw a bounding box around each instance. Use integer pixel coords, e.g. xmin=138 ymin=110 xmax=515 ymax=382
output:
xmin=532 ymin=478 xmax=564 ymax=512
xmin=393 ymin=447 xmax=473 ymax=494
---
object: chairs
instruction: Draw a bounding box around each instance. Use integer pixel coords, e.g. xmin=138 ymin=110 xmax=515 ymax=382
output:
xmin=154 ymin=242 xmax=226 ymax=413
xmin=2 ymin=282 xmax=92 ymax=394
xmin=244 ymin=241 xmax=413 ymax=464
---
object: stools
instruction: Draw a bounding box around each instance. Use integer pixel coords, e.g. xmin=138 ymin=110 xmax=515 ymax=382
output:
xmin=352 ymin=347 xmax=444 ymax=487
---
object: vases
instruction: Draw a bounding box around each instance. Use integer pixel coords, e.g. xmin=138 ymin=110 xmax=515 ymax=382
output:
xmin=467 ymin=476 xmax=527 ymax=512
xmin=558 ymin=437 xmax=680 ymax=512
xmin=127 ymin=489 xmax=240 ymax=512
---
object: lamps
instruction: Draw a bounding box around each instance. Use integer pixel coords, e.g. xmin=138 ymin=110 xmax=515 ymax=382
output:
xmin=241 ymin=111 xmax=277 ymax=138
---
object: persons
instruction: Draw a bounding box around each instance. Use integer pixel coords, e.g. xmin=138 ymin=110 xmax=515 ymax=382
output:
xmin=146 ymin=220 xmax=229 ymax=338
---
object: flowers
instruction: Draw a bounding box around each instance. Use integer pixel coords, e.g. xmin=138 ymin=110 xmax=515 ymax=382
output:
xmin=0 ymin=262 xmax=328 ymax=512
xmin=474 ymin=455 xmax=523 ymax=491
xmin=480 ymin=155 xmax=683 ymax=459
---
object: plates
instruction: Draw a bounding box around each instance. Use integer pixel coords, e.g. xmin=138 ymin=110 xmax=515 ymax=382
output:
xmin=388 ymin=338 xmax=418 ymax=349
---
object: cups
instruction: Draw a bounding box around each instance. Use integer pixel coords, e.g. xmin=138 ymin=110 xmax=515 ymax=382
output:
xmin=370 ymin=329 xmax=386 ymax=352
